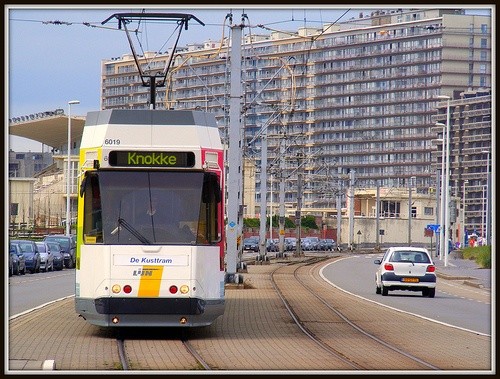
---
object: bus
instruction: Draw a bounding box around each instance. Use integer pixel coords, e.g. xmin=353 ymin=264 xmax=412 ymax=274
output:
xmin=74 ymin=109 xmax=225 ymax=328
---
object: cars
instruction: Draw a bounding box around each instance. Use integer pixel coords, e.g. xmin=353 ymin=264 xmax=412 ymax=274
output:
xmin=374 ymin=247 xmax=436 ymax=298
xmin=242 ymin=237 xmax=338 ymax=252
xmin=10 ymin=235 xmax=77 ymax=275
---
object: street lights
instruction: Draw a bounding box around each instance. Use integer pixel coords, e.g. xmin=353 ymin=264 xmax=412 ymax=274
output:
xmin=66 ymin=101 xmax=81 ymax=235
xmin=431 ymin=122 xmax=445 ymax=262
xmin=433 ymin=94 xmax=450 ymax=267
